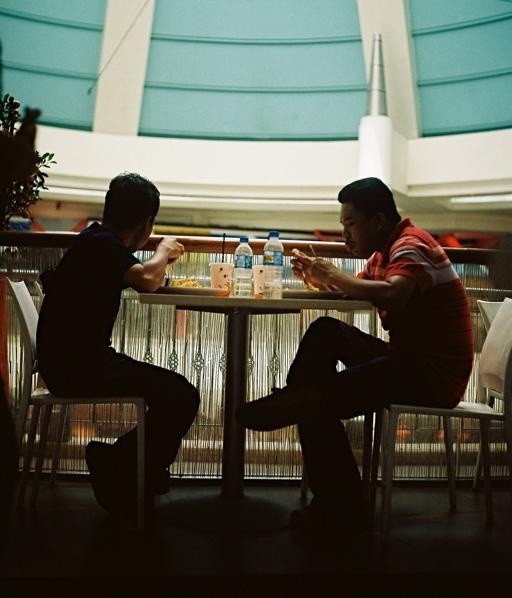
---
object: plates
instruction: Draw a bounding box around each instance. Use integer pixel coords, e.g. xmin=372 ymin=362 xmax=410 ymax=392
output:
xmin=163 ymin=284 xmax=229 ymax=296
xmin=281 ymin=287 xmax=348 ymax=300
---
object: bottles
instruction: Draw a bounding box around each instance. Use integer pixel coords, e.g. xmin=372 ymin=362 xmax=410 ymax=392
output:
xmin=263 ymin=231 xmax=284 ymax=301
xmin=232 ymin=237 xmax=254 ymax=299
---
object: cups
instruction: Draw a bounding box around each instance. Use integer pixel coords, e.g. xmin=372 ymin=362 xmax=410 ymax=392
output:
xmin=208 ymin=262 xmax=234 ymax=291
xmin=252 ymin=265 xmax=276 ymax=300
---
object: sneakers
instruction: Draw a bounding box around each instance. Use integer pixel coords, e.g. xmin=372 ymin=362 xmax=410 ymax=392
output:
xmin=287 ymin=498 xmax=370 ymax=533
xmin=235 ymin=382 xmax=323 ymax=431
xmin=84 ymin=440 xmax=156 ymax=526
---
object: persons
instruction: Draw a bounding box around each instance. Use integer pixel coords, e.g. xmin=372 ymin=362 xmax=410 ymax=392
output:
xmin=41 ymin=171 xmax=202 ymax=517
xmin=235 ymin=176 xmax=475 ymax=530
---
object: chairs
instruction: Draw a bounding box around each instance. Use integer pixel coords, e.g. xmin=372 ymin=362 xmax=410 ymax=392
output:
xmin=3 ymin=279 xmax=149 ymax=524
xmin=364 ymin=296 xmax=511 ymax=554
